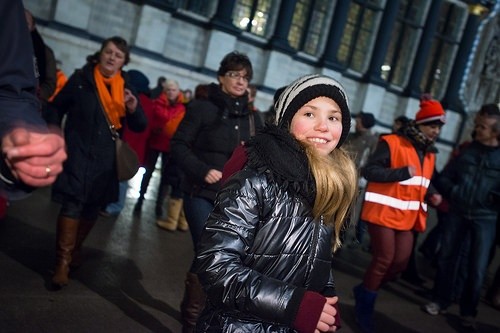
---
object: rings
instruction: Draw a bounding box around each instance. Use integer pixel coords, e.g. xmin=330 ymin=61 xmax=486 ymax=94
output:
xmin=45 ymin=165 xmax=51 ymax=177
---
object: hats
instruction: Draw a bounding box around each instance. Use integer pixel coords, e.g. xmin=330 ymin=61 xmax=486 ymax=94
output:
xmin=415 ymin=94 xmax=445 ymax=124
xmin=274 ymin=74 xmax=351 ymax=149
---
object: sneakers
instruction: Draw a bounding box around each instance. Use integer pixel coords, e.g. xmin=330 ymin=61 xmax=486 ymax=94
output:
xmin=424 ymin=301 xmax=448 ymax=315
xmin=459 ymin=316 xmax=476 ymax=330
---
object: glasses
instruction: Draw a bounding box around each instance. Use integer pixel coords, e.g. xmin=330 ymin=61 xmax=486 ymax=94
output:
xmin=226 ymin=71 xmax=249 ymax=82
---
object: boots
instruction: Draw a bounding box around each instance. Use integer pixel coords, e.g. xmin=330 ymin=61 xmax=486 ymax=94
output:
xmin=52 ymin=214 xmax=81 ymax=284
xmin=157 ymin=198 xmax=184 ymax=232
xmin=180 ymin=272 xmax=206 ymax=333
xmin=177 ymin=211 xmax=190 ymax=232
xmin=352 ymin=280 xmax=378 ymax=332
xmin=71 ymin=219 xmax=97 ymax=265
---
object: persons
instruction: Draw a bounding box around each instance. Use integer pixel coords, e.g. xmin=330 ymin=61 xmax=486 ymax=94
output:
xmin=340 ymin=93 xmax=500 ymax=333
xmin=182 ymin=73 xmax=359 ymax=333
xmin=0 ymin=0 xmax=268 ymax=333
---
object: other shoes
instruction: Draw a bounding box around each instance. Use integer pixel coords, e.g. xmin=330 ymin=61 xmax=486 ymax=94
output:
xmin=139 ymin=193 xmax=144 ymax=200
xmin=99 ymin=209 xmax=110 ymax=217
